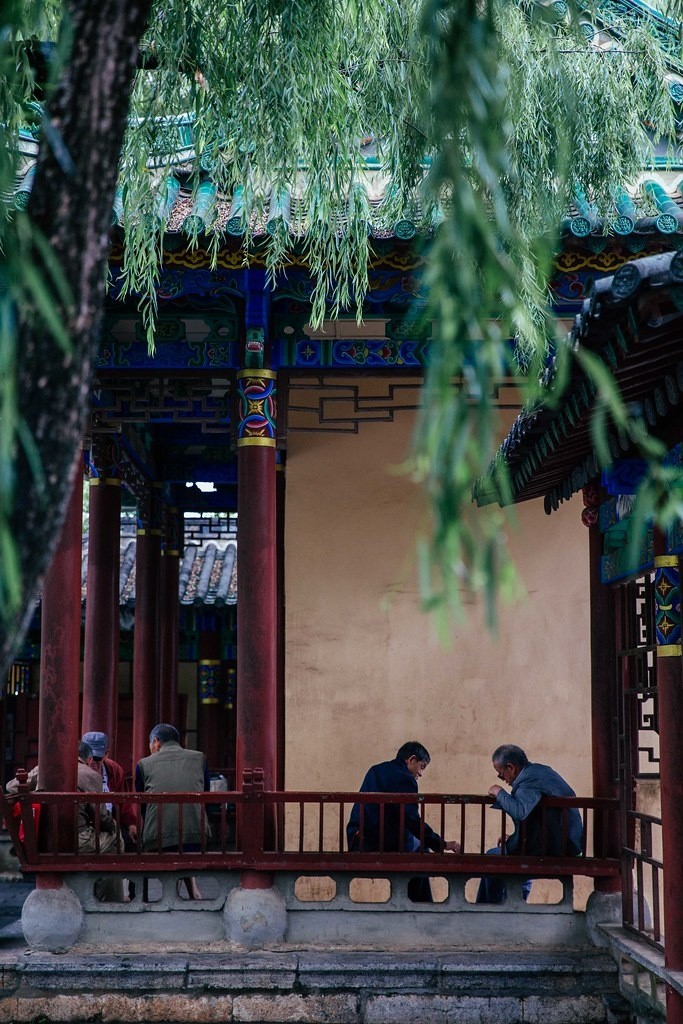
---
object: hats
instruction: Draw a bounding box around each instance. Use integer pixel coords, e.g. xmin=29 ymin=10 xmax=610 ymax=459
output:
xmin=81 ymin=731 xmax=108 ymax=757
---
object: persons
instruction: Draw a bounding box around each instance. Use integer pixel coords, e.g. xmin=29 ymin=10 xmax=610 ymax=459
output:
xmin=348 ymin=742 xmax=460 ymax=854
xmin=6 ymin=723 xmax=212 ymax=899
xmin=476 ymin=744 xmax=583 ymax=904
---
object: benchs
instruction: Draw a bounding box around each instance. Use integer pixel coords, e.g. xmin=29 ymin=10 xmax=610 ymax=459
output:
xmin=0 ymin=769 xmax=616 ymax=901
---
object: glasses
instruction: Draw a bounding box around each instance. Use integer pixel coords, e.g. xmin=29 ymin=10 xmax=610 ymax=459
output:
xmin=93 ymin=748 xmax=108 ymax=762
xmin=497 ymin=762 xmax=506 ymax=781
